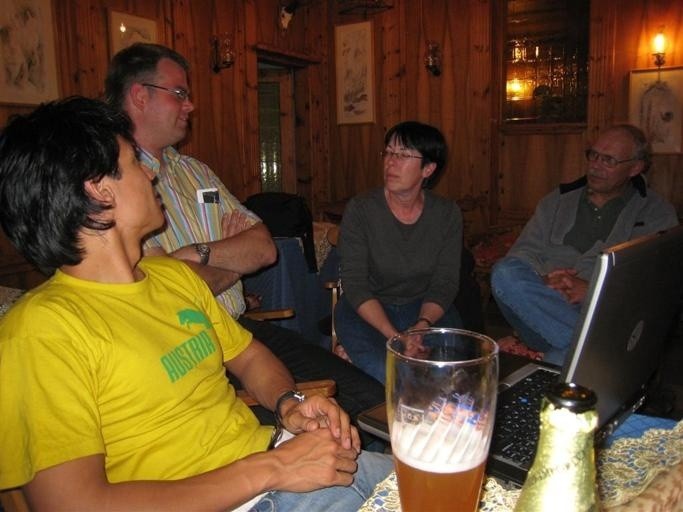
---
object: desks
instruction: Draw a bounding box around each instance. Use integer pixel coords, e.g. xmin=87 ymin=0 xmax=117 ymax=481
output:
xmin=356 ymin=413 xmax=683 ymax=512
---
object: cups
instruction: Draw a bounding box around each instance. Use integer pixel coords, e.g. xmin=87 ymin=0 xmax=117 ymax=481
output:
xmin=384 ymin=327 xmax=500 ymax=512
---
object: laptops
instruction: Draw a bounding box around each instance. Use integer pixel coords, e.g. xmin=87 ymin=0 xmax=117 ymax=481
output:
xmin=354 ymin=223 xmax=682 ymax=488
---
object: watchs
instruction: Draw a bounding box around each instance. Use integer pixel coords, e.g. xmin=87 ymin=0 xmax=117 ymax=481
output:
xmin=191 ymin=243 xmax=210 ymax=265
xmin=274 ymin=390 xmax=307 ymax=428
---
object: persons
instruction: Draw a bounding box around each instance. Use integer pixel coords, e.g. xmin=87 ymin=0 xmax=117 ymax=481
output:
xmin=490 ymin=125 xmax=679 ymax=367
xmin=333 ymin=123 xmax=469 ymax=453
xmin=105 ymin=43 xmax=384 ymax=448
xmin=0 ymin=97 xmax=395 ymax=512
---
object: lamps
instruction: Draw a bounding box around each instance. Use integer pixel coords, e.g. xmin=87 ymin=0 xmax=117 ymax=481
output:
xmin=424 ymin=40 xmax=440 ymax=75
xmin=511 ymin=42 xmax=527 ymax=65
xmin=212 ymin=30 xmax=235 ymax=71
xmin=651 ymin=33 xmax=666 ymax=67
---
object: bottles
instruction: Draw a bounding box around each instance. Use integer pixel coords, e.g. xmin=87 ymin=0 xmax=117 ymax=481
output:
xmin=514 ymin=381 xmax=599 ymax=512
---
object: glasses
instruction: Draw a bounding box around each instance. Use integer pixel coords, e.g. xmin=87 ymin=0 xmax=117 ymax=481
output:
xmin=584 ymin=149 xmax=639 ymax=168
xmin=141 ymin=83 xmax=191 ymax=100
xmin=380 ymin=150 xmax=424 ymax=160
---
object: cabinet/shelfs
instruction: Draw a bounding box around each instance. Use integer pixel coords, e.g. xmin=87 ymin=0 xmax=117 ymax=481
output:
xmin=509 ymin=37 xmax=587 ymax=122
xmin=242 ymin=307 xmax=294 ymax=324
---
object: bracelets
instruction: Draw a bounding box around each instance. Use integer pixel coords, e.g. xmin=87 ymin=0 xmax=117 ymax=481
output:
xmin=415 ymin=317 xmax=433 ymax=328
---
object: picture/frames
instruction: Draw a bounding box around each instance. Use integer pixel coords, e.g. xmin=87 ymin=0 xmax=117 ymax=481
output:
xmin=0 ymin=0 xmax=63 ymax=107
xmin=332 ymin=19 xmax=378 ymax=128
xmin=107 ymin=7 xmax=165 ymax=61
xmin=628 ymin=66 xmax=683 ymax=156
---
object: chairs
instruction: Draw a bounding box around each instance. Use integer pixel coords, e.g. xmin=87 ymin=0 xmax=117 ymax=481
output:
xmin=0 ymin=379 xmax=336 ymax=512
xmin=324 ymin=226 xmax=352 ymax=364
